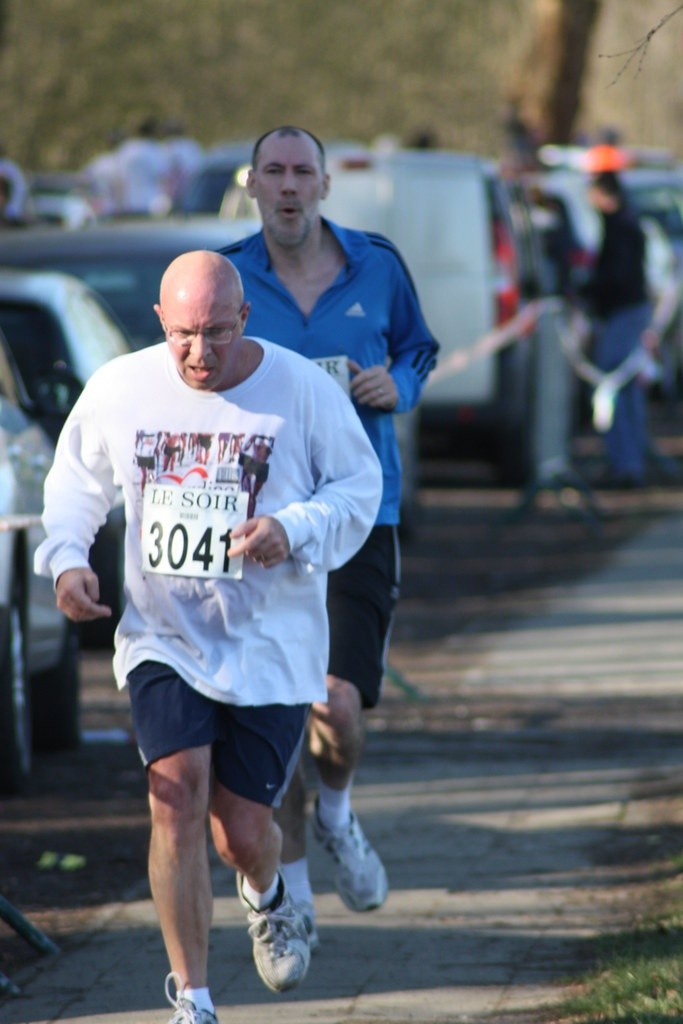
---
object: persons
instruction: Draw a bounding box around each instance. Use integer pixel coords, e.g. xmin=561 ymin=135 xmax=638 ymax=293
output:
xmin=1 ymin=111 xmax=206 ymax=228
xmin=584 ymin=170 xmax=679 ymax=488
xmin=503 ymin=95 xmax=621 ymax=172
xmin=212 ymin=127 xmax=440 ymax=945
xmin=32 ymin=251 xmax=384 ymax=1024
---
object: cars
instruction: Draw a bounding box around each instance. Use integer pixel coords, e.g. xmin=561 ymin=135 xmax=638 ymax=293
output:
xmin=0 ymin=131 xmax=683 ymax=794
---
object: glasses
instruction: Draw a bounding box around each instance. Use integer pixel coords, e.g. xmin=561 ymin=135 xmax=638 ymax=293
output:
xmin=159 ymin=310 xmax=243 ymax=345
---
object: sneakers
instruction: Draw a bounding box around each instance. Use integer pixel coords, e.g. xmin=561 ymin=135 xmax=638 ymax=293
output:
xmin=288 ymin=875 xmax=321 ymax=953
xmin=310 ymin=795 xmax=389 ymax=913
xmin=235 ymin=870 xmax=311 ymax=993
xmin=164 ymin=971 xmax=219 ymax=1024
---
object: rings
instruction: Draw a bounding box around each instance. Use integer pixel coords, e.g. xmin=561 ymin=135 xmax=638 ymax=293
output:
xmin=377 ymin=387 xmax=384 ymax=397
